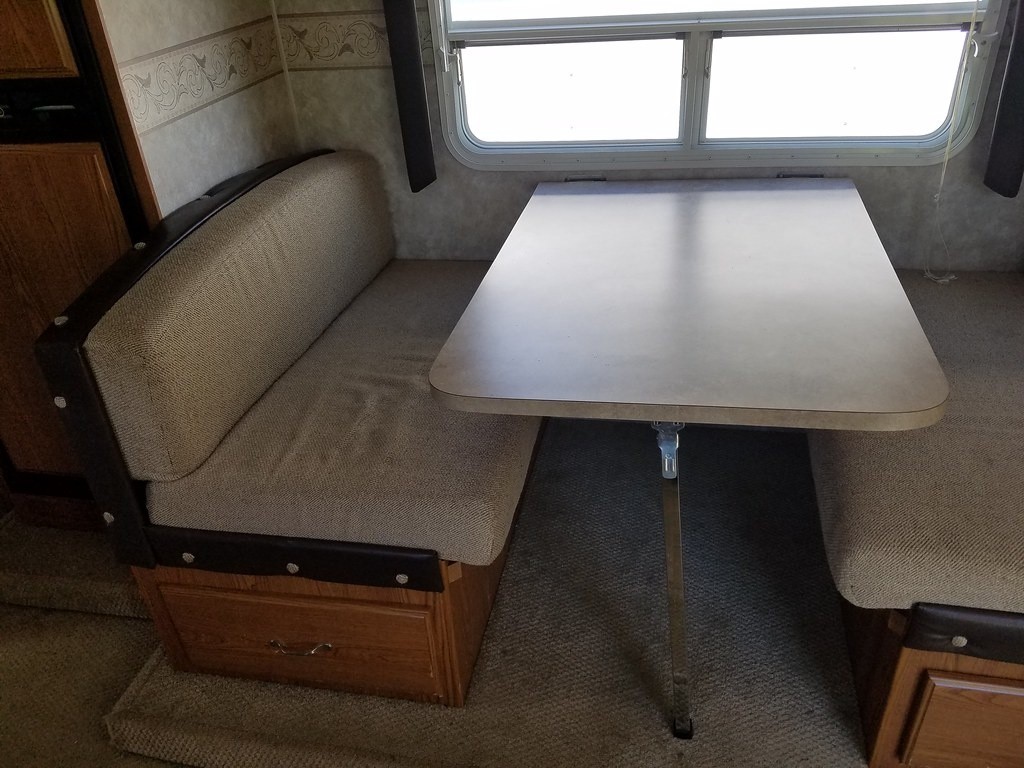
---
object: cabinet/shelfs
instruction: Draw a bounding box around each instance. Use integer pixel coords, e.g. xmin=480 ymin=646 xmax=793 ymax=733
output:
xmin=0 ymin=1 xmax=162 ymax=491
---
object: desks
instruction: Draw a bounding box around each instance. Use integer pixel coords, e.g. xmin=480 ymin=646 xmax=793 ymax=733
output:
xmin=427 ymin=179 xmax=950 ymax=741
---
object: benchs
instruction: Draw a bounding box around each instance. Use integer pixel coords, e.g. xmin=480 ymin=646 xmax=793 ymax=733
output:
xmin=32 ymin=148 xmax=548 ymax=706
xmin=806 ymin=266 xmax=1024 ymax=768
